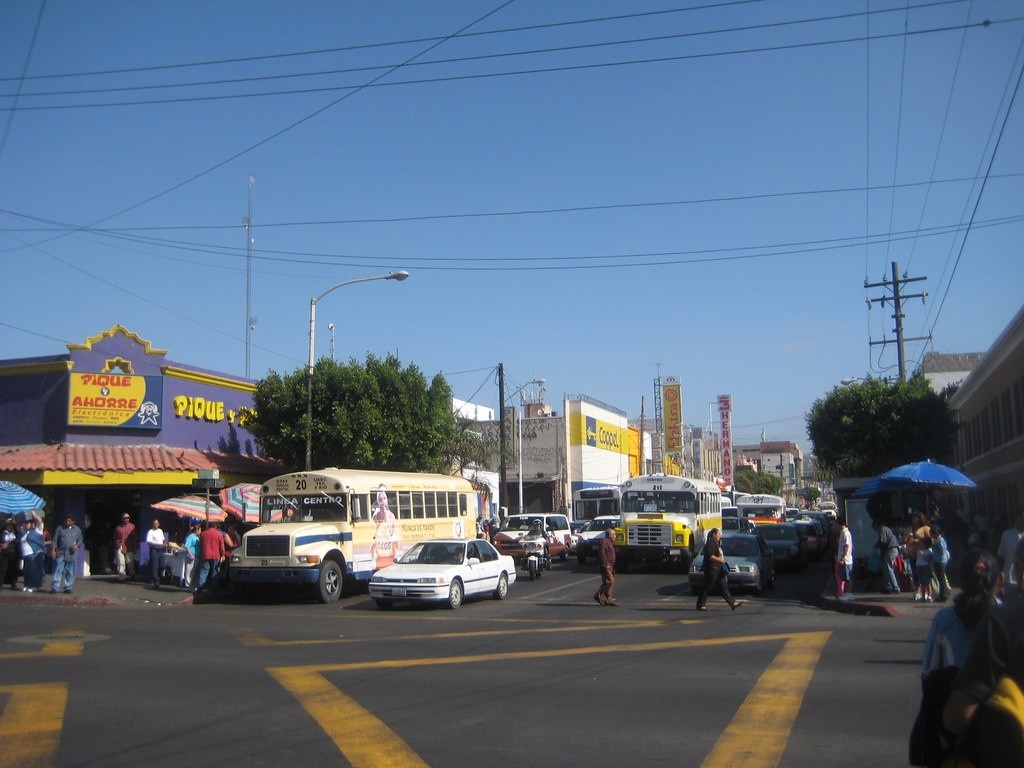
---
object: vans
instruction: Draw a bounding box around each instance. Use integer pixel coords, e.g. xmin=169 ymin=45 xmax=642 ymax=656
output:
xmin=493 ymin=513 xmax=572 ymax=565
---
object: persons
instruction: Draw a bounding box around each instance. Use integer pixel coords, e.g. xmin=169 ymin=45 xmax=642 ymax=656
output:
xmin=112 ymin=513 xmax=137 ymax=583
xmin=833 ymin=518 xmax=854 ymax=600
xmin=920 ymin=548 xmax=1005 ymax=698
xmin=521 ymin=520 xmax=553 ymax=570
xmin=146 ymin=518 xmax=166 ymax=592
xmin=696 ymin=527 xmax=744 ymax=611
xmin=0 ymin=510 xmax=47 ymax=593
xmin=872 ymin=489 xmax=1024 ymax=603
xmin=475 ymin=515 xmax=500 ymax=543
xmin=594 ymin=529 xmax=617 ymax=606
xmin=940 ymin=537 xmax=1024 ymax=768
xmin=184 ymin=521 xmax=241 ymax=592
xmin=370 ymin=484 xmax=399 ymax=570
xmin=51 ymin=514 xmax=83 ymax=595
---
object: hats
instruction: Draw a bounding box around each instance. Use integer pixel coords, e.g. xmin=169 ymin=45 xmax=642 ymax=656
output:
xmin=122 ymin=513 xmax=130 ymax=519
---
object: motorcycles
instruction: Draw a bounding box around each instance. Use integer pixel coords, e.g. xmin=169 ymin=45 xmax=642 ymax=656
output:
xmin=518 ymin=533 xmax=552 ymax=581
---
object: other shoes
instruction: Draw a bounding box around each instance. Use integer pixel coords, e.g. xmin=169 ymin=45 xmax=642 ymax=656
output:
xmin=64 ymin=589 xmax=72 ymax=593
xmin=594 ymin=595 xmax=617 ymax=606
xmin=696 ymin=605 xmax=708 ymax=611
xmin=840 ymin=593 xmax=856 ymax=601
xmin=11 ymin=585 xmax=21 ymax=591
xmin=914 ymin=593 xmax=950 ymax=602
xmin=50 ymin=590 xmax=58 ymax=594
xmin=731 ymin=602 xmax=743 ymax=609
xmin=23 ymin=587 xmax=39 ymax=593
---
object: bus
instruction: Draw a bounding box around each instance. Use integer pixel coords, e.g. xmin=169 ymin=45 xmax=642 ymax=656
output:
xmin=228 ymin=466 xmax=490 ymax=604
xmin=735 ymin=493 xmax=787 ymax=528
xmin=573 ymin=486 xmax=622 ymax=531
xmin=614 ymin=473 xmax=722 ymax=572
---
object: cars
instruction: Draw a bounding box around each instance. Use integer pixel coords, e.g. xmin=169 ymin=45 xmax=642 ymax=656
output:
xmin=576 ymin=515 xmax=620 ymax=565
xmin=368 ymin=538 xmax=517 ymax=610
xmin=571 ymin=521 xmax=590 ymax=556
xmin=689 ymin=534 xmax=776 ymax=596
xmin=752 ymin=525 xmax=810 ymax=573
xmin=722 ymin=501 xmax=837 ymax=563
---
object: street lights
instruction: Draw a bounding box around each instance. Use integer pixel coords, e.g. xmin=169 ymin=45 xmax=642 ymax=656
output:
xmin=305 ymin=270 xmax=410 ymax=470
xmin=499 ymin=379 xmax=546 ymax=520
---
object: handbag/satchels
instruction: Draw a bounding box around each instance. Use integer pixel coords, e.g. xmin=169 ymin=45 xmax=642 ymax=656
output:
xmin=867 ymin=546 xmax=882 ymax=572
xmin=722 ymin=561 xmax=730 ymax=575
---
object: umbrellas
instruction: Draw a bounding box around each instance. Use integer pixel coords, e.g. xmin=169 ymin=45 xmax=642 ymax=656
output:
xmin=149 ymin=494 xmax=228 ymax=533
xmin=218 ymin=483 xmax=294 ymax=527
xmin=850 ymin=472 xmax=914 ymax=498
xmin=0 ymin=480 xmax=48 ymax=516
xmin=881 ymin=457 xmax=978 ymax=517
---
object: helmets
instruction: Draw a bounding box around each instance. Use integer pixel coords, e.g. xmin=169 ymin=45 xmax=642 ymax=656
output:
xmin=533 ymin=519 xmax=543 ymax=529
